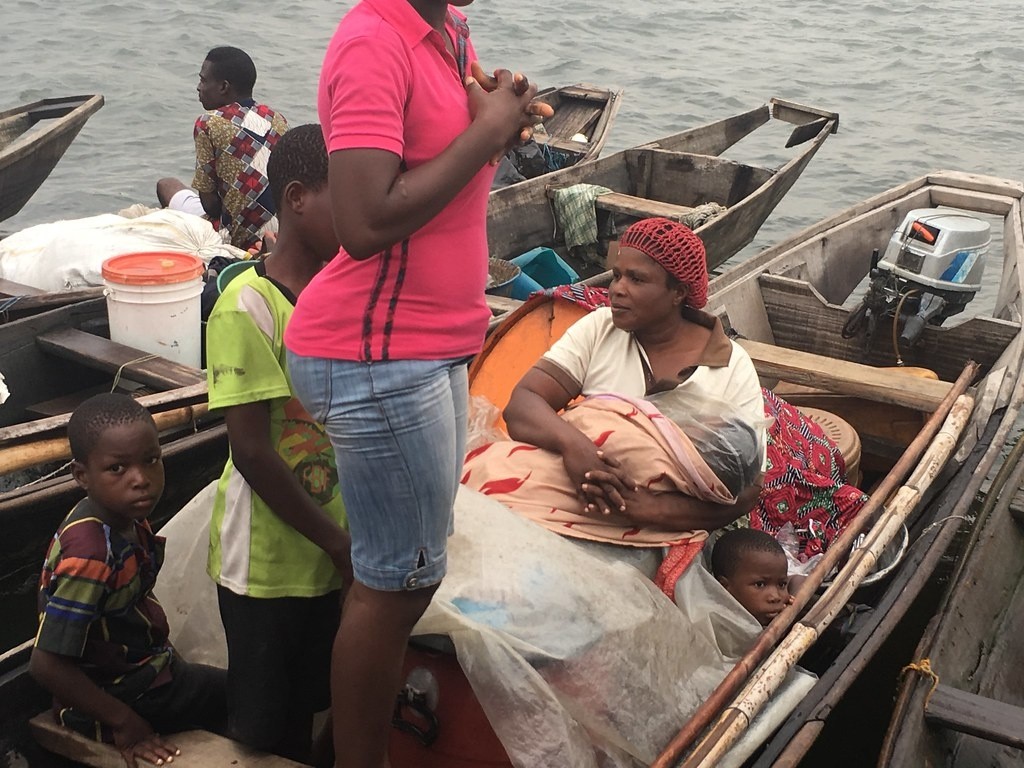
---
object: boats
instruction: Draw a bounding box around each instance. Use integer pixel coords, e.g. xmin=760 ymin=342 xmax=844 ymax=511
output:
xmin=2 ymin=168 xmax=1024 ymax=767
xmin=1 ymin=78 xmax=627 ymax=388
xmin=877 ymin=428 xmax=1023 ymax=767
xmin=1 ymin=91 xmax=841 ymax=503
xmin=1 ymin=93 xmax=106 ymax=224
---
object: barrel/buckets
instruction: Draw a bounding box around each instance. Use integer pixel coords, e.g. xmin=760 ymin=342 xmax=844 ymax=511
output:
xmin=815 ymin=505 xmax=908 ymax=588
xmin=217 ymin=261 xmax=260 ymax=295
xmin=103 ymin=252 xmax=205 ymax=391
xmin=485 ymin=258 xmax=521 ymax=297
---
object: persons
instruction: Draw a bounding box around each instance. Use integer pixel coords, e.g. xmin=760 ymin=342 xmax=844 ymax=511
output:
xmin=283 ymin=0 xmax=554 ymax=768
xmin=156 ymin=47 xmax=291 ymax=254
xmin=410 ymin=217 xmax=814 ymax=657
xmin=205 ymin=123 xmax=353 ymax=762
xmin=30 ymin=393 xmax=235 ymax=768
